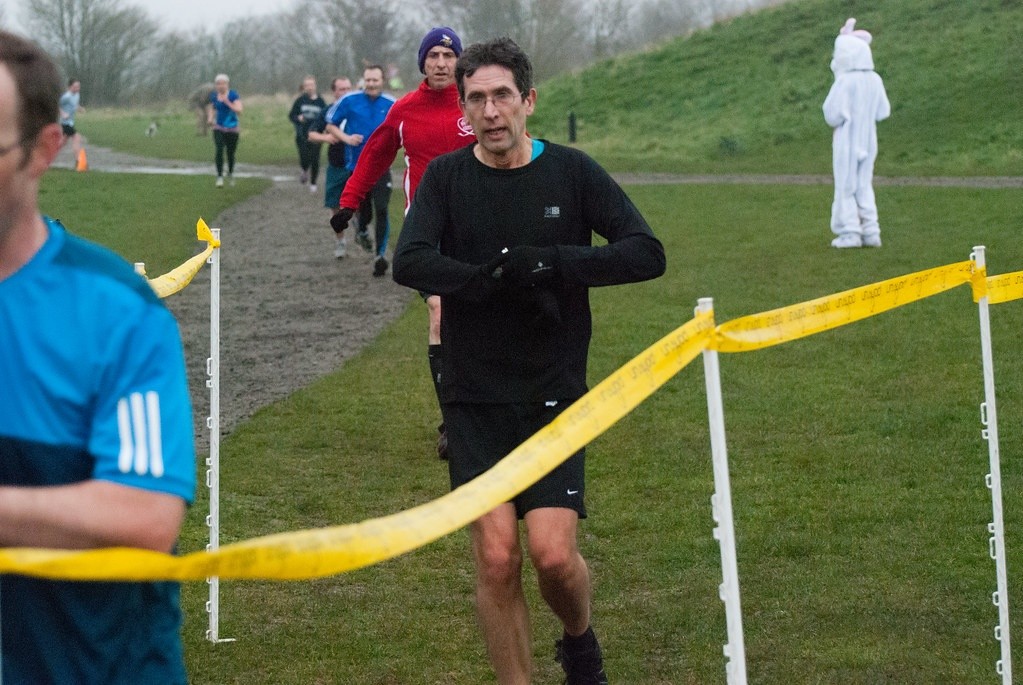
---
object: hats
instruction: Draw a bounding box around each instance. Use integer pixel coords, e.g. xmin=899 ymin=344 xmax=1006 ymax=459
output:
xmin=418 ymin=27 xmax=462 ymax=73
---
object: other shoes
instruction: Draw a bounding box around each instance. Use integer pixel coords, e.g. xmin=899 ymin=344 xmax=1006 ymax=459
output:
xmin=373 ymin=256 xmax=388 ymax=276
xmin=310 ymin=185 xmax=318 ymax=192
xmin=438 ymin=432 xmax=448 ymax=459
xmin=300 ymin=170 xmax=307 ymax=183
xmin=336 ymin=244 xmax=347 ymax=258
xmin=216 ymin=178 xmax=224 ymax=188
xmin=228 ymin=177 xmax=235 ymax=185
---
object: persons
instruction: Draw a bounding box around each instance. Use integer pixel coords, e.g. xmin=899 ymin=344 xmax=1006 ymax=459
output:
xmin=393 ymin=37 xmax=666 ymax=685
xmin=206 ymin=74 xmax=243 ymax=187
xmin=59 ymin=78 xmax=80 ymax=164
xmin=331 ymin=27 xmax=531 ymax=460
xmin=308 ymin=75 xmax=373 ymax=254
xmin=187 ymin=83 xmax=215 ymax=135
xmin=0 ymin=29 xmax=196 ymax=685
xmin=325 ymin=65 xmax=397 ymax=277
xmin=289 ymin=77 xmax=328 ymax=193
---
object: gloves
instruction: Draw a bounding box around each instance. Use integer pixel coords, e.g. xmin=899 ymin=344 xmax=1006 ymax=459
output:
xmin=331 ymin=207 xmax=353 ymax=233
xmin=487 ymin=244 xmax=559 ymax=286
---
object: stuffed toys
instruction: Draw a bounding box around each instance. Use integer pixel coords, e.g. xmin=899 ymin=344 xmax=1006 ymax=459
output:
xmin=821 ymin=18 xmax=891 ymax=249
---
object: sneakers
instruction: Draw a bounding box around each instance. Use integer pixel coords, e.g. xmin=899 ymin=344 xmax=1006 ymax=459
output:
xmin=561 ymin=635 xmax=609 ymax=685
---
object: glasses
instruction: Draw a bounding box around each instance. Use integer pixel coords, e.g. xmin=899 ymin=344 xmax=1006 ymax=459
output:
xmin=461 ymin=91 xmax=524 ymax=110
